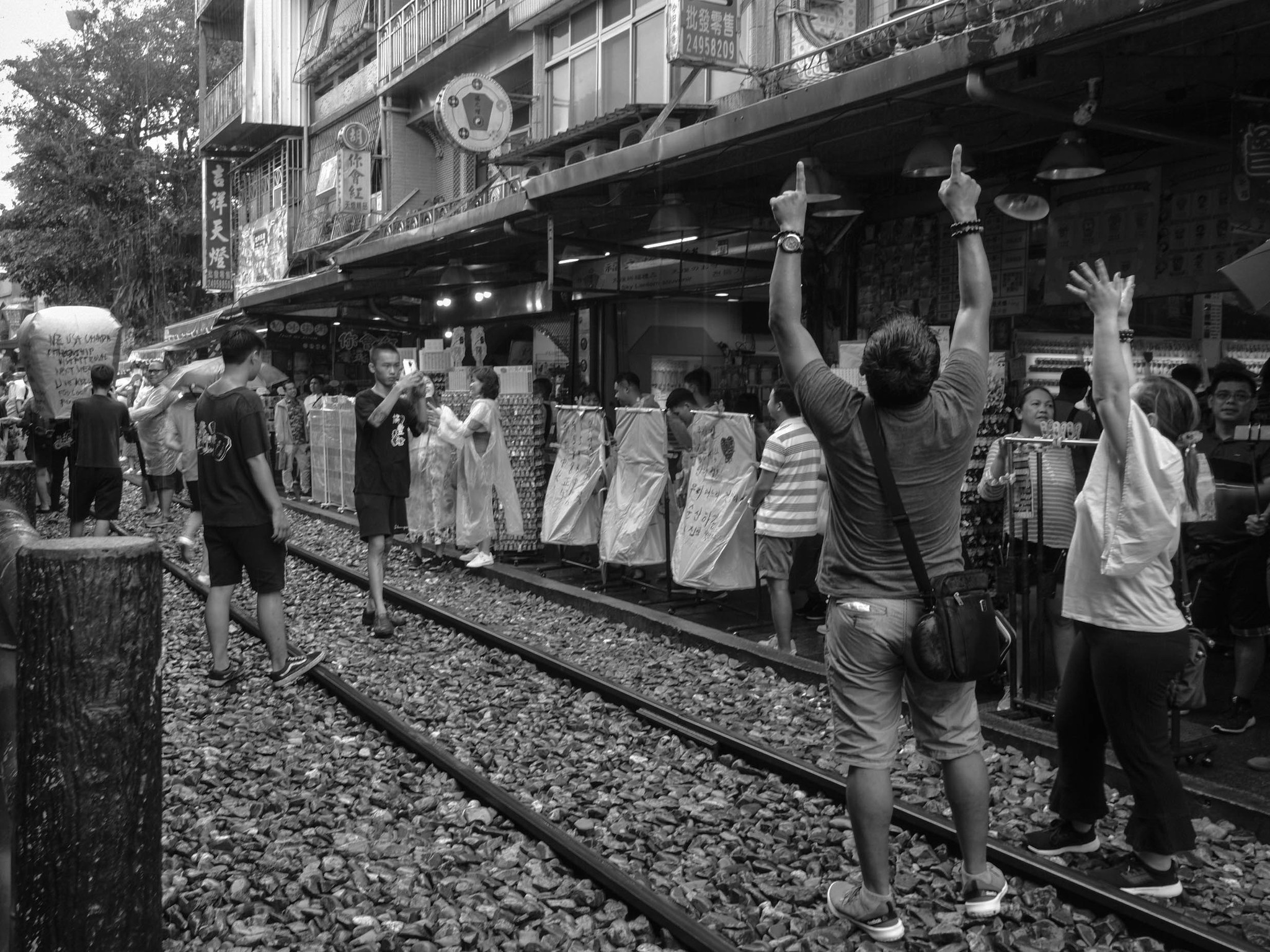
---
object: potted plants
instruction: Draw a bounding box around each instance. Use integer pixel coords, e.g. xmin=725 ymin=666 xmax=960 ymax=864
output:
xmin=308 ymin=12 xmax=378 ymax=79
xmin=826 ymin=37 xmax=866 ymax=73
xmin=931 ymin=0 xmax=968 ymax=37
xmin=863 ymin=17 xmax=897 ymax=61
xmin=889 ymin=0 xmax=935 ymax=50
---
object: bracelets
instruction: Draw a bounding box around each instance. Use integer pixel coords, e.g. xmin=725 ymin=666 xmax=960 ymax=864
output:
xmin=772 ymin=231 xmax=805 ymax=240
xmin=949 ymin=217 xmax=981 ymax=229
xmin=747 ymin=498 xmax=756 ymax=509
xmin=1120 ymin=338 xmax=1133 ymax=343
xmin=1118 ymin=328 xmax=1134 ymax=333
xmin=950 ymin=227 xmax=983 ymax=239
xmin=1120 ymin=333 xmax=1133 ymax=338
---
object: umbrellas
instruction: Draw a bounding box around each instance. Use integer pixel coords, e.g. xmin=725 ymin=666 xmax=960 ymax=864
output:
xmin=158 ymin=355 xmax=289 ymax=390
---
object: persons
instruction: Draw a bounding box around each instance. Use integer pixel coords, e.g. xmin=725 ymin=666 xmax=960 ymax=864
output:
xmin=977 ymin=358 xmax=1270 ymax=772
xmin=353 ymin=343 xmax=427 ymax=638
xmin=194 ymin=327 xmax=324 ymax=688
xmin=404 ymin=367 xmax=524 ymax=567
xmin=1022 ymin=257 xmax=1204 ymax=899
xmin=532 ymin=367 xmax=830 ymax=656
xmin=0 ymin=361 xmax=358 ymax=585
xmin=767 ymin=145 xmax=1009 ymax=942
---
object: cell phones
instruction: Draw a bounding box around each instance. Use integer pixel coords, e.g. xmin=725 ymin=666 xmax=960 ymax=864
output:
xmin=403 ymin=359 xmax=416 ymax=377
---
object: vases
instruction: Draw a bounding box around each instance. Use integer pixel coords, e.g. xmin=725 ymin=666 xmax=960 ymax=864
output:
xmin=993 ymin=0 xmax=1019 ymax=19
xmin=965 ymin=0 xmax=992 ymax=28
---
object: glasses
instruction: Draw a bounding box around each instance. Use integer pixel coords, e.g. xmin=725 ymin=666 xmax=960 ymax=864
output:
xmin=149 ymin=369 xmax=164 ymax=375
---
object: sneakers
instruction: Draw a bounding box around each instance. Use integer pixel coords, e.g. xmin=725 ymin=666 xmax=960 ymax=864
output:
xmin=961 ymin=861 xmax=1008 ymax=918
xmin=373 ymin=614 xmax=393 ymax=637
xmin=827 ymin=880 xmax=904 ymax=942
xmin=1210 ymin=706 xmax=1257 ymax=732
xmin=1024 ymin=818 xmax=1100 ymax=854
xmin=270 ymin=650 xmax=325 ymax=686
xmin=362 ymin=608 xmax=406 ymax=626
xmin=1085 ymin=852 xmax=1183 ymax=897
xmin=206 ymin=658 xmax=246 ymax=686
xmin=760 ymin=633 xmax=797 ymax=657
xmin=816 ymin=625 xmax=826 ymax=634
xmin=466 ymin=551 xmax=494 ymax=568
xmin=428 ymin=555 xmax=450 ymax=570
xmin=459 ymin=546 xmax=480 ymax=562
xmin=409 ymin=555 xmax=425 ymax=569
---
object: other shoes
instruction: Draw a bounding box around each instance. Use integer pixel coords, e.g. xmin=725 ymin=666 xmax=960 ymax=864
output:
xmin=805 ymin=606 xmax=827 ymax=620
xmin=1248 ymin=757 xmax=1270 ymax=769
xmin=198 ymin=574 xmax=213 ymax=586
xmin=179 ymin=535 xmax=196 ymax=565
xmin=146 ymin=514 xmax=174 ymax=527
xmin=287 ymin=492 xmax=296 ymax=501
xmin=301 ymin=490 xmax=312 ymax=498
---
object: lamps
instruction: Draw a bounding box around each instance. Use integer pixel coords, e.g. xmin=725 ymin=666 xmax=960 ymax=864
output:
xmin=811 ymin=172 xmax=865 ymax=218
xmin=1036 ymin=124 xmax=1107 ymax=180
xmin=992 ymin=165 xmax=1051 ymax=222
xmin=649 ymin=192 xmax=701 ymax=231
xmin=779 ymin=143 xmax=842 ymax=204
xmin=900 ymin=111 xmax=978 ymax=178
xmin=438 ymin=257 xmax=475 ymax=286
xmin=560 ymin=229 xmax=606 ymax=262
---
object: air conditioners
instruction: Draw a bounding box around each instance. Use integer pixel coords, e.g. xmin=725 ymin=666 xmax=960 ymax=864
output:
xmin=365 ymin=191 xmax=382 ymax=232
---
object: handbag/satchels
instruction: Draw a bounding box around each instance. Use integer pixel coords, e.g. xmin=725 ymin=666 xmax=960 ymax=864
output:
xmin=275 ymin=449 xmax=286 ymax=471
xmin=929 ymin=567 xmax=1008 ymax=680
xmin=1168 ymin=622 xmax=1214 ymax=709
xmin=912 ymin=613 xmax=948 ymax=682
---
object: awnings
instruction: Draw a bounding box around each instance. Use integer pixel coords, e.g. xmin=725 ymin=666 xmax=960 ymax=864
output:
xmin=117 ymin=302 xmax=242 ymax=376
xmin=241 ymin=0 xmax=1270 ymax=324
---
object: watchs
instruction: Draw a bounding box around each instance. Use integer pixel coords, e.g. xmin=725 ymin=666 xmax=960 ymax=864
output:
xmin=777 ymin=235 xmax=805 ymax=252
